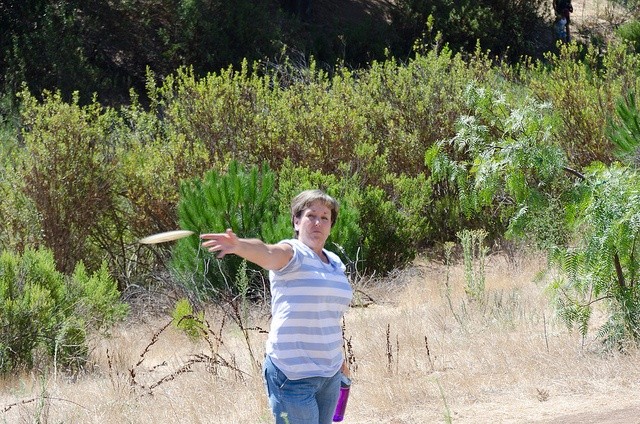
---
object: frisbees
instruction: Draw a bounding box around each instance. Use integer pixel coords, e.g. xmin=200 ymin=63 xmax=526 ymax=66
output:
xmin=139 ymin=230 xmax=193 ymax=245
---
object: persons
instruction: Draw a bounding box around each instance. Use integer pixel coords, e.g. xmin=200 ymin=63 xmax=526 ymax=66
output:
xmin=199 ymin=189 xmax=354 ymax=424
